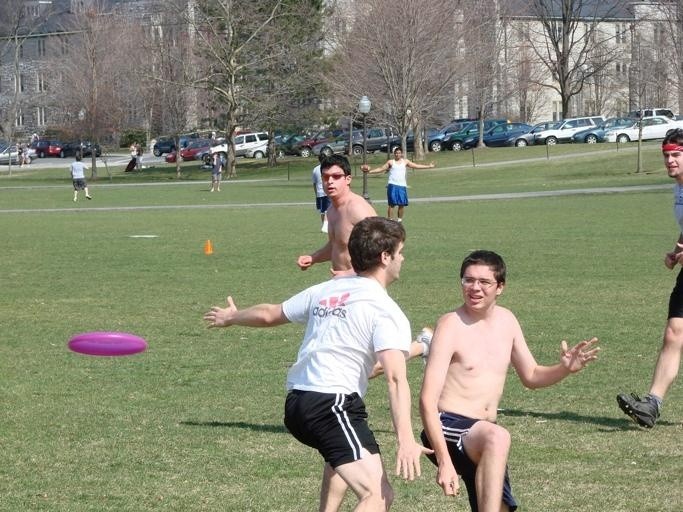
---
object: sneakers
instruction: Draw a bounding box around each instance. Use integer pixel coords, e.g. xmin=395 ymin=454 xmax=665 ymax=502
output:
xmin=416 ymin=325 xmax=435 ymax=366
xmin=616 ymin=391 xmax=663 ymax=430
xmin=84 ymin=195 xmax=93 ymax=200
xmin=320 ymin=223 xmax=329 ymax=234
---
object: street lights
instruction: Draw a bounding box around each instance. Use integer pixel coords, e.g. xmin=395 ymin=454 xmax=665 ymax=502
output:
xmin=358 ymin=95 xmax=373 ymax=203
xmin=75 ymin=108 xmax=85 ymax=164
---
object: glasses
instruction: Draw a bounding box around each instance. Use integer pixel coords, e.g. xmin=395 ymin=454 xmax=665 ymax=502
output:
xmin=459 ymin=276 xmax=500 ymax=288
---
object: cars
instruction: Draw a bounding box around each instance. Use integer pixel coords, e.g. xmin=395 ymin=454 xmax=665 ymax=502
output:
xmin=0 ymin=137 xmax=100 ymax=168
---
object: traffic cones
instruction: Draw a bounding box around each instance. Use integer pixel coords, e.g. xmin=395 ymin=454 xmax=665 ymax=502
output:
xmin=203 ymin=239 xmax=213 ymax=255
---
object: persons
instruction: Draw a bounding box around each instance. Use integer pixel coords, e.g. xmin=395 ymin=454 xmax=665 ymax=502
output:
xmin=129 ymin=141 xmax=138 ymax=170
xmin=418 ymin=250 xmax=602 ymax=512
xmin=209 ymin=152 xmax=223 ymax=193
xmin=70 ymin=155 xmax=92 ymax=202
xmin=18 ymin=131 xmax=40 ymax=168
xmin=203 ymin=215 xmax=435 ymax=512
xmin=363 ymin=147 xmax=440 ymax=223
xmin=310 ymin=154 xmax=333 ymax=234
xmin=296 ymin=154 xmax=436 ymax=380
xmin=616 ymin=126 xmax=683 ymax=430
xmin=135 ymin=143 xmax=143 ymax=172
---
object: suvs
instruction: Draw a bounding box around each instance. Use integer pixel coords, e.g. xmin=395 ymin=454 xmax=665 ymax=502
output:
xmin=151 ymin=127 xmax=268 ymax=167
